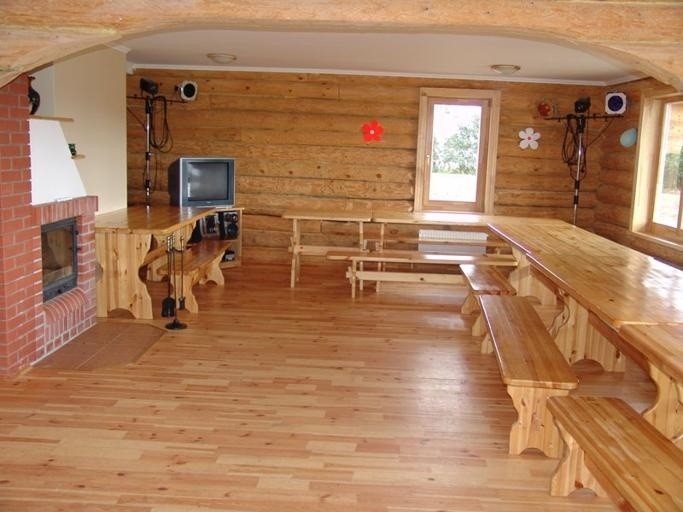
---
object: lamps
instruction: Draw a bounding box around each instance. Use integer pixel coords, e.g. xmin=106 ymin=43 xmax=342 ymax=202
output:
xmin=208 ymin=53 xmax=236 ymax=64
xmin=492 ymin=64 xmax=520 ymax=74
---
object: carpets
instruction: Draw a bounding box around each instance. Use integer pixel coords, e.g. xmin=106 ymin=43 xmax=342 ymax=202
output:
xmin=35 ymin=322 xmax=168 ymax=370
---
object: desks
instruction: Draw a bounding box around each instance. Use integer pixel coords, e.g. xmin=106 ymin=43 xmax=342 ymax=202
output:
xmin=488 ymin=222 xmax=593 ymax=296
xmin=622 ymin=323 xmax=683 ymax=442
xmin=372 ymin=209 xmax=564 ymax=292
xmin=526 ymin=253 xmax=683 ymax=372
xmin=282 ymin=209 xmax=373 ymax=291
xmin=95 ymin=204 xmax=216 ymax=319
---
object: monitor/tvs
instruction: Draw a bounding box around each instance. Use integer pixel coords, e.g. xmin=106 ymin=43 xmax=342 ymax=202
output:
xmin=167 ymin=157 xmax=235 ymax=208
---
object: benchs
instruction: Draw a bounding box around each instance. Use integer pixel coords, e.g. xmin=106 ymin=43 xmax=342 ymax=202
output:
xmin=324 ymin=249 xmax=518 ymax=298
xmin=460 ymin=264 xmax=516 ymax=314
xmin=156 ymin=239 xmax=232 ymax=313
xmin=548 ymin=393 xmax=683 ymax=512
xmin=473 ymin=294 xmax=578 ymax=459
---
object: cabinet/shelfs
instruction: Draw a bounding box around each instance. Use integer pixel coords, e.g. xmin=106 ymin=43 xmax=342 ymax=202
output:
xmin=195 ymin=206 xmax=245 ymax=269
